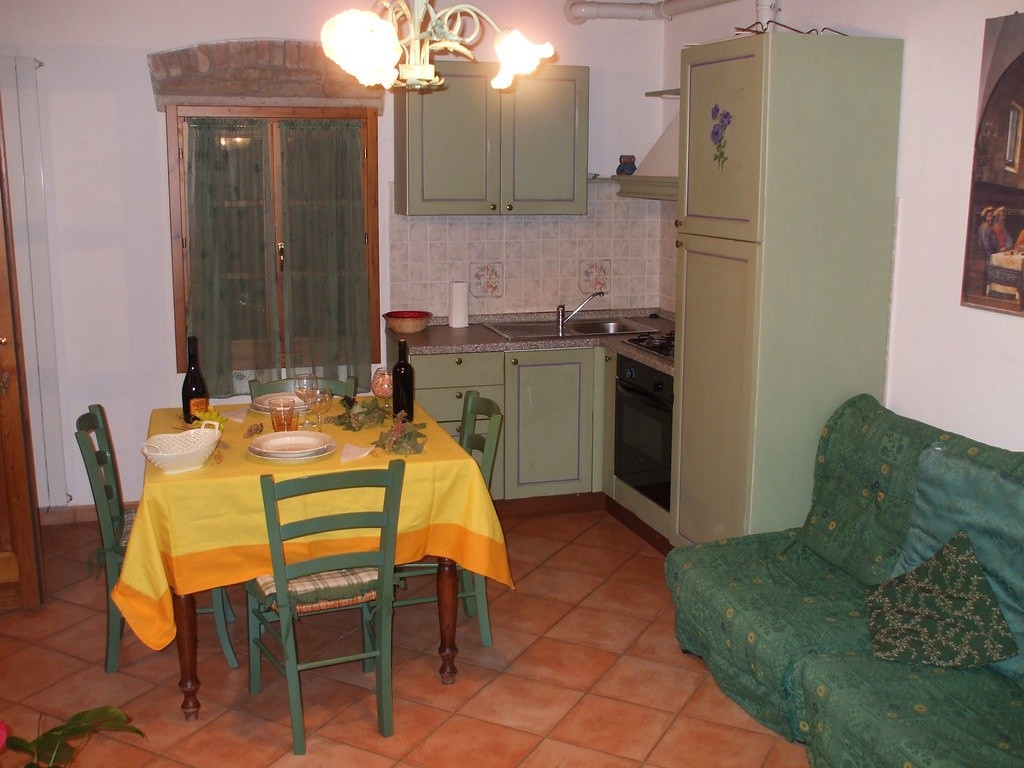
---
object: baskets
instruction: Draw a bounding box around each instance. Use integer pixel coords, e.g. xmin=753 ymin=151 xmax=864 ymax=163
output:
xmin=140 ymin=419 xmax=222 ymax=475
xmin=381 ymin=310 xmax=433 ymax=334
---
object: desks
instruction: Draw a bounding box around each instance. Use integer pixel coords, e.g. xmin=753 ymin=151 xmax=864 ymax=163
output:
xmin=112 ymin=397 xmax=514 ymax=721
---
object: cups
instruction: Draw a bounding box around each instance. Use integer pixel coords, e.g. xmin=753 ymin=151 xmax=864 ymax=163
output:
xmin=274 ymin=409 xmax=298 ymax=433
xmin=269 ymin=399 xmax=295 ymax=428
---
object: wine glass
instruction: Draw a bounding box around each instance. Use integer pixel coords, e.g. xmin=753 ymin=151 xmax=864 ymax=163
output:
xmin=307 ymin=386 xmax=333 ymax=433
xmin=293 ymin=373 xmax=318 ymax=428
xmin=371 ymin=367 xmax=392 ymax=417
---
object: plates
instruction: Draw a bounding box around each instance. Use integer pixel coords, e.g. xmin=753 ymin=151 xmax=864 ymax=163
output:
xmin=247 ymin=443 xmax=337 ymax=462
xmin=250 ymin=403 xmax=312 ymax=416
xmin=253 ymin=392 xmax=307 ymax=408
xmin=249 ymin=431 xmax=333 ymax=455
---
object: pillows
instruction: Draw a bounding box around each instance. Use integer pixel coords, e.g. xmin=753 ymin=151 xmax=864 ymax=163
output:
xmin=890 ymin=447 xmax=1024 ymax=673
xmin=866 ymin=534 xmax=1019 ymax=667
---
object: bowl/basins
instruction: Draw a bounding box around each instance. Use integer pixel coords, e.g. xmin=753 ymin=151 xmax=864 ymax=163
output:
xmin=382 ymin=310 xmax=433 ymax=333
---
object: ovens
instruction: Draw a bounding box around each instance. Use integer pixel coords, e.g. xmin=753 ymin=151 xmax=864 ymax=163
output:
xmin=614 ymin=356 xmax=674 ymax=512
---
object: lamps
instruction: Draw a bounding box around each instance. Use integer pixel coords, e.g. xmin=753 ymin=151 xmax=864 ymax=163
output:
xmin=320 ymin=0 xmax=557 ymax=92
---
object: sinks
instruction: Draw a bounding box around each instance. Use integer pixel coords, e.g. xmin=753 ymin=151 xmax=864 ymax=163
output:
xmin=481 ymin=320 xmax=577 ymax=340
xmin=566 ymin=318 xmax=661 ymax=336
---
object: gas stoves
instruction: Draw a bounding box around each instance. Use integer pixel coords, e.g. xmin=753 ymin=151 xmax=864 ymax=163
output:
xmin=622 ymin=329 xmax=675 ymax=361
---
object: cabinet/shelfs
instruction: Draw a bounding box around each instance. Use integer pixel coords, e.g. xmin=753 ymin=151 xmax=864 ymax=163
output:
xmin=503 ymin=349 xmax=593 ymax=498
xmin=410 ymin=355 xmax=504 ymax=501
xmin=394 ymin=58 xmax=588 ymax=215
xmin=670 ymin=27 xmax=904 ymax=544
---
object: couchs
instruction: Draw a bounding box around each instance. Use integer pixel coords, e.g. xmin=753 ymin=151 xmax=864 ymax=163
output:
xmin=660 ymin=394 xmax=1024 ymax=768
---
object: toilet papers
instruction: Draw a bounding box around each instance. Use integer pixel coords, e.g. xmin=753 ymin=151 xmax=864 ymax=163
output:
xmin=448 ymin=281 xmax=469 ymax=328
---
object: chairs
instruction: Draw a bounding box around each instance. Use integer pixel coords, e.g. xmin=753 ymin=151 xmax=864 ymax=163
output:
xmin=74 ymin=404 xmax=239 ymax=673
xmin=243 ymin=458 xmax=407 ymax=755
xmin=391 ymin=390 xmax=503 ymax=647
xmin=246 ymin=376 xmax=358 ymax=400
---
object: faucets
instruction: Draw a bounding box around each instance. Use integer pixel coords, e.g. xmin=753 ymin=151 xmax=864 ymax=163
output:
xmin=558 ymin=291 xmax=604 ymax=337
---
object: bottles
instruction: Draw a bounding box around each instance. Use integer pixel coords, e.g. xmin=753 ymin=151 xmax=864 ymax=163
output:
xmin=181 ymin=336 xmax=209 ymax=422
xmin=392 ymin=339 xmax=414 ymax=423
